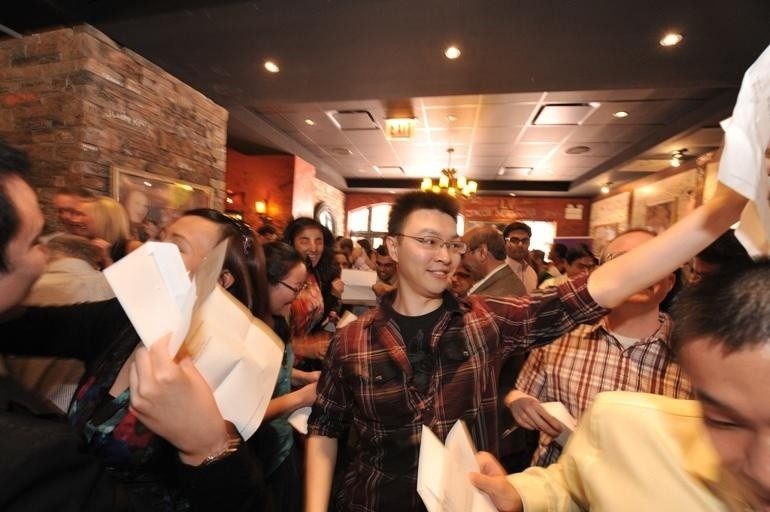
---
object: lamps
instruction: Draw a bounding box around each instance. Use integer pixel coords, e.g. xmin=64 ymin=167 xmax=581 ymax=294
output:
xmin=255 ymin=199 xmax=273 ymax=224
xmin=601 ymin=182 xmax=612 ymax=195
xmin=670 ymin=154 xmax=695 ymax=167
xmin=564 ymin=204 xmax=584 ymax=221
xmin=421 ymin=149 xmax=478 ymax=198
xmin=382 ymin=114 xmax=417 ymax=138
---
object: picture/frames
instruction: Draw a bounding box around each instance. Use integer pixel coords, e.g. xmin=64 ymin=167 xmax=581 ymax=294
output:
xmin=109 ymin=162 xmax=216 ymax=242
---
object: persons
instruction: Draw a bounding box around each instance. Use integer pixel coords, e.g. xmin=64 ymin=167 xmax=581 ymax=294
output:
xmin=2 ymin=148 xmax=769 ymax=511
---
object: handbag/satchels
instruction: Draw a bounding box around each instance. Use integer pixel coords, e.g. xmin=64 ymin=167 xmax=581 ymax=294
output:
xmin=81 ymin=387 xmax=191 ymax=512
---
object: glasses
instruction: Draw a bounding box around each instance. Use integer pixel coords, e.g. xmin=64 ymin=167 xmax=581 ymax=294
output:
xmin=387 ymin=232 xmax=467 ymax=254
xmin=231 ymin=219 xmax=254 ymax=255
xmin=504 ymin=237 xmax=530 ymax=244
xmin=606 ymin=250 xmax=627 ymax=260
xmin=277 ymin=280 xmax=307 ymax=295
